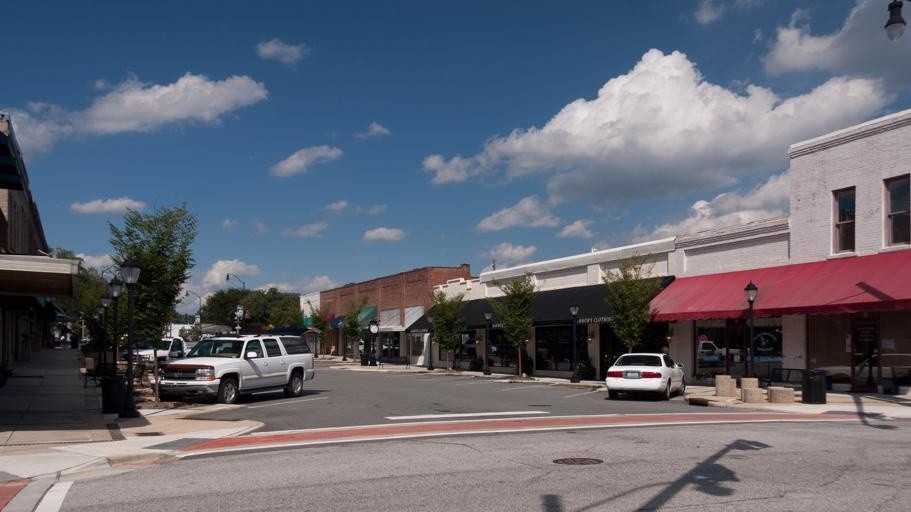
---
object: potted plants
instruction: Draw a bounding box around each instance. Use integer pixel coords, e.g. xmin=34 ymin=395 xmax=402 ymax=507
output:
xmin=575 ymin=359 xmax=595 ymax=380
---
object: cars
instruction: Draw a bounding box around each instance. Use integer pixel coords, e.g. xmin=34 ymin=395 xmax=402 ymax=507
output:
xmin=121 ymin=338 xmax=185 ymax=358
xmin=697 ymin=341 xmax=722 ymax=363
xmin=605 ymin=353 xmax=685 ymax=400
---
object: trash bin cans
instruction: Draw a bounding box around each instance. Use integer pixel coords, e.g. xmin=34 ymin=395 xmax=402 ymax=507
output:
xmin=100 ymin=376 xmax=126 ymax=413
xmin=360 ymin=354 xmax=369 ymax=365
xmin=802 ymin=370 xmax=826 ymax=404
xmin=370 ymin=355 xmax=376 ymax=366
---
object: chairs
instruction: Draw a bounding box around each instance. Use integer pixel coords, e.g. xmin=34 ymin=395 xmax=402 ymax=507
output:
xmin=80 ymin=357 xmax=102 ymax=388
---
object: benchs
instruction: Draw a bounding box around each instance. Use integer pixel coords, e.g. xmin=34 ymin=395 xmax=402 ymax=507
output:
xmin=377 ymin=357 xmax=410 ymax=370
xmin=768 ymin=368 xmax=804 ymax=387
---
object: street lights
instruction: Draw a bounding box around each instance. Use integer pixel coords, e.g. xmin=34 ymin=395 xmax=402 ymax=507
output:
xmin=482 ymin=313 xmax=492 ymax=375
xmin=185 ymin=291 xmax=201 ymax=308
xmin=744 ymin=280 xmax=757 ymax=378
xmin=226 ymin=272 xmax=245 ymax=290
xmin=569 ymin=304 xmax=580 ymax=383
xmin=91 ymin=258 xmax=140 ymax=419
xmin=369 ymin=320 xmax=379 ymax=366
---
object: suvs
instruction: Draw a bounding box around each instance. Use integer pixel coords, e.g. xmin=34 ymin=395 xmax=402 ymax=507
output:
xmin=157 ymin=334 xmax=314 ymax=403
